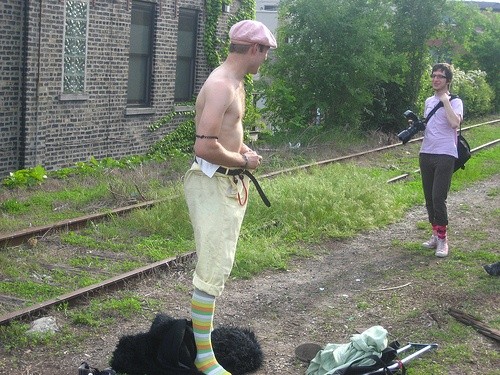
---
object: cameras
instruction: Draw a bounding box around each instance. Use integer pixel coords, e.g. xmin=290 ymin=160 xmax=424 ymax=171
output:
xmin=396 ymin=109 xmax=425 ymax=145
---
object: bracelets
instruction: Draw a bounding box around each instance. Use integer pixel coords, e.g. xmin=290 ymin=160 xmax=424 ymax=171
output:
xmin=240 ymin=154 xmax=249 ymax=169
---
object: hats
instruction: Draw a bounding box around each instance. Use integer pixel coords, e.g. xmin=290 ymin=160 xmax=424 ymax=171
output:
xmin=228 ymin=20 xmax=278 ymax=50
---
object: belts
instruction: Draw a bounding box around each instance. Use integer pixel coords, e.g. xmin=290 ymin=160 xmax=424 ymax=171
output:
xmin=194 ymin=157 xmax=273 ymax=210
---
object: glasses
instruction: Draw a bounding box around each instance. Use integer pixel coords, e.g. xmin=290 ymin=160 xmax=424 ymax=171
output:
xmin=430 ymin=73 xmax=449 ymax=80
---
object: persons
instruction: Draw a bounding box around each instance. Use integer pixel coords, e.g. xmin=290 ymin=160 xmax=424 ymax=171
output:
xmin=407 ymin=63 xmax=464 ymax=258
xmin=182 ymin=19 xmax=278 ymax=375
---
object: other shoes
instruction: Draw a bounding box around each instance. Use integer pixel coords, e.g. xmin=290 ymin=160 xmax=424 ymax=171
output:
xmin=422 ymin=235 xmax=438 ymax=248
xmin=435 ymin=237 xmax=449 ymax=258
xmin=483 ymin=262 xmax=500 ymax=276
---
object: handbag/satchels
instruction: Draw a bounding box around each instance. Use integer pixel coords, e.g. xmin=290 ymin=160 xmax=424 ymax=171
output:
xmin=453 ymin=135 xmax=471 ymax=174
